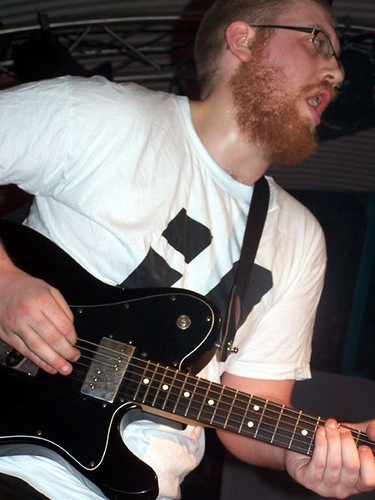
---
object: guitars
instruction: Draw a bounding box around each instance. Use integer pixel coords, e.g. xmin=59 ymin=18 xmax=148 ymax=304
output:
xmin=1 ymin=219 xmax=374 ymax=500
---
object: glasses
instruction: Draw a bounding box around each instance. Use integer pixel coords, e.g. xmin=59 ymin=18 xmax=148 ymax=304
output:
xmin=248 ymin=24 xmax=345 ymax=89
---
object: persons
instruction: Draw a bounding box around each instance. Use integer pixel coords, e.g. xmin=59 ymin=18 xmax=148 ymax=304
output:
xmin=0 ymin=0 xmax=375 ymax=500
